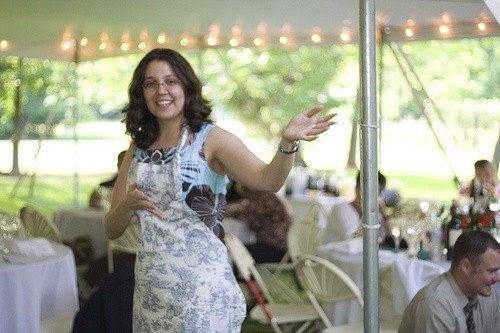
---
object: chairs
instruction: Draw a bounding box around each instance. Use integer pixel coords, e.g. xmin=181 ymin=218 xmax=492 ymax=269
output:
xmin=19 ymin=205 xmax=99 ymax=304
xmin=220 ymin=202 xmax=401 ymax=333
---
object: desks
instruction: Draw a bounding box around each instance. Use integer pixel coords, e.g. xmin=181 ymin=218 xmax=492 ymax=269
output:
xmin=320 ymin=236 xmax=452 ymax=325
xmin=0 ymin=241 xmax=79 ymax=333
xmin=52 ymin=206 xmax=108 ymax=258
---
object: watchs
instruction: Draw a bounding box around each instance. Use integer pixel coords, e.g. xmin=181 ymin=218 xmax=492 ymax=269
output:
xmin=278 ymin=139 xmax=300 ymax=154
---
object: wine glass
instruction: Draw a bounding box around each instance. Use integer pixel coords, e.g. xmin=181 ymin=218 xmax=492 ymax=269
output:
xmin=388 ymin=200 xmax=440 ymax=252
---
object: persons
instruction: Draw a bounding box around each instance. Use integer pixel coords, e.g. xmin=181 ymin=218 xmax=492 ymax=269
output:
xmin=223 ymin=178 xmax=293 ymax=278
xmin=460 ymin=160 xmax=500 ymax=196
xmin=104 ymin=48 xmax=338 ymax=333
xmin=321 ymin=168 xmax=392 ymax=244
xmin=397 ymin=230 xmax=500 ymax=333
xmin=89 ymin=151 xmax=126 ymax=209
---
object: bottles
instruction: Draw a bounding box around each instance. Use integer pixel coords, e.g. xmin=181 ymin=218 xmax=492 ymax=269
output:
xmin=0 ymin=213 xmax=22 ymax=237
xmin=447 ymin=206 xmax=464 ymax=259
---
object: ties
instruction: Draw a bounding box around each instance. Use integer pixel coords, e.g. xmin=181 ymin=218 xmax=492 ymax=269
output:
xmin=464 ymin=304 xmax=477 ymax=333
